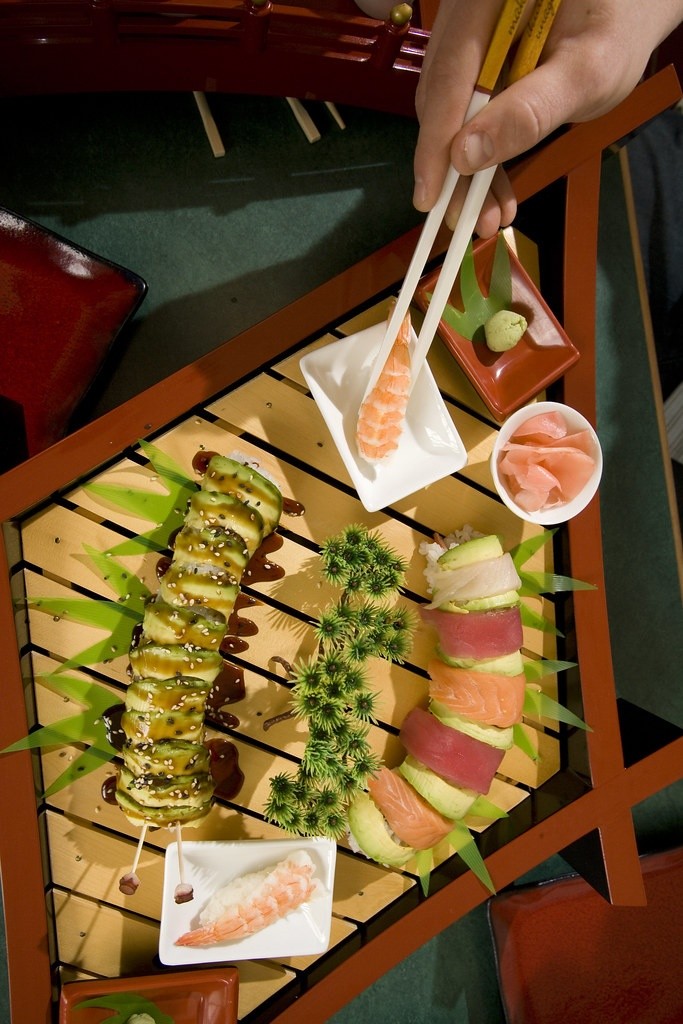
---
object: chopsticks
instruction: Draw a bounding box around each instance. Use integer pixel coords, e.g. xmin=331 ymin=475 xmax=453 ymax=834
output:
xmin=193 ymin=90 xmax=346 ymax=158
xmin=356 ymin=0 xmax=563 ymax=414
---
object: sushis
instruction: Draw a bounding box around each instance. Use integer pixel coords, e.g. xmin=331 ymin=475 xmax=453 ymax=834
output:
xmin=114 ymin=449 xmax=283 ymax=829
xmin=343 ymin=527 xmax=526 ymax=869
xmin=176 ymin=850 xmax=316 ymax=949
xmin=356 ymin=301 xmax=415 ymax=461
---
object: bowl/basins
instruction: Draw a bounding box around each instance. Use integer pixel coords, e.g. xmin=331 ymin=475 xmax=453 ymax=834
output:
xmin=490 ymin=401 xmax=603 ymax=525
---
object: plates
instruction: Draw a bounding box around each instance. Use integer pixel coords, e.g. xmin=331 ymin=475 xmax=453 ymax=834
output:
xmin=411 ymin=230 xmax=581 ymax=420
xmin=159 ymin=836 xmax=337 ymax=966
xmin=59 ymin=967 xmax=240 ymax=1024
xmin=299 ymin=318 xmax=468 ymax=514
xmin=1 ymin=207 xmax=148 ymax=475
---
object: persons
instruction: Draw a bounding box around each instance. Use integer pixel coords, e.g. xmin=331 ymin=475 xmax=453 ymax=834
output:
xmin=413 ymin=1 xmax=683 ymax=534
